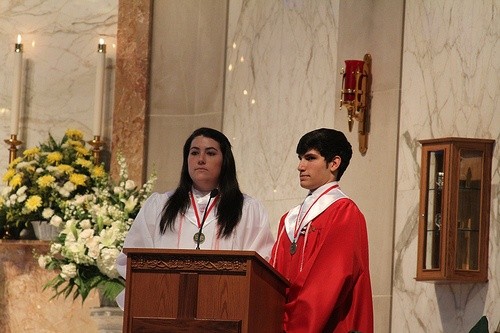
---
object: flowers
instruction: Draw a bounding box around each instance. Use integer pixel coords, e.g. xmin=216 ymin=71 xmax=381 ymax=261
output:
xmin=30 ymin=146 xmax=158 ymax=307
xmin=1 ymin=128 xmax=108 ymax=241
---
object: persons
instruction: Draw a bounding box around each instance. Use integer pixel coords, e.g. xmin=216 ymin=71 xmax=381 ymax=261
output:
xmin=268 ymin=128 xmax=374 ymax=333
xmin=114 ymin=126 xmax=274 ymax=313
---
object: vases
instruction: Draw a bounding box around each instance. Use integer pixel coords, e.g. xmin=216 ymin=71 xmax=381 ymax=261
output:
xmin=27 ymin=220 xmax=64 ymax=242
xmin=95 ymin=283 xmax=119 ymax=307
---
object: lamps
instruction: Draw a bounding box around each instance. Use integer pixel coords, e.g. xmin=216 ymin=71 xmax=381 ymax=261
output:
xmin=4 ymin=33 xmax=25 ymax=169
xmin=85 ymin=37 xmax=107 ymax=167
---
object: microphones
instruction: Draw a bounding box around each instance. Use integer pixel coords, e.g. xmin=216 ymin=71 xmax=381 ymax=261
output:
xmin=196 ymin=188 xmax=219 ymax=249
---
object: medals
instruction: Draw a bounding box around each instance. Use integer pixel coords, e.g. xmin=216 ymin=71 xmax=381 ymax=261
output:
xmin=193 ymin=231 xmax=206 ymax=243
xmin=289 ymin=239 xmax=297 ymax=255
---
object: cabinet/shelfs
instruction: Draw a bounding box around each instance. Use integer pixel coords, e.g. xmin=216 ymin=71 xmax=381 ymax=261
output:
xmin=413 ymin=136 xmax=495 ymax=284
